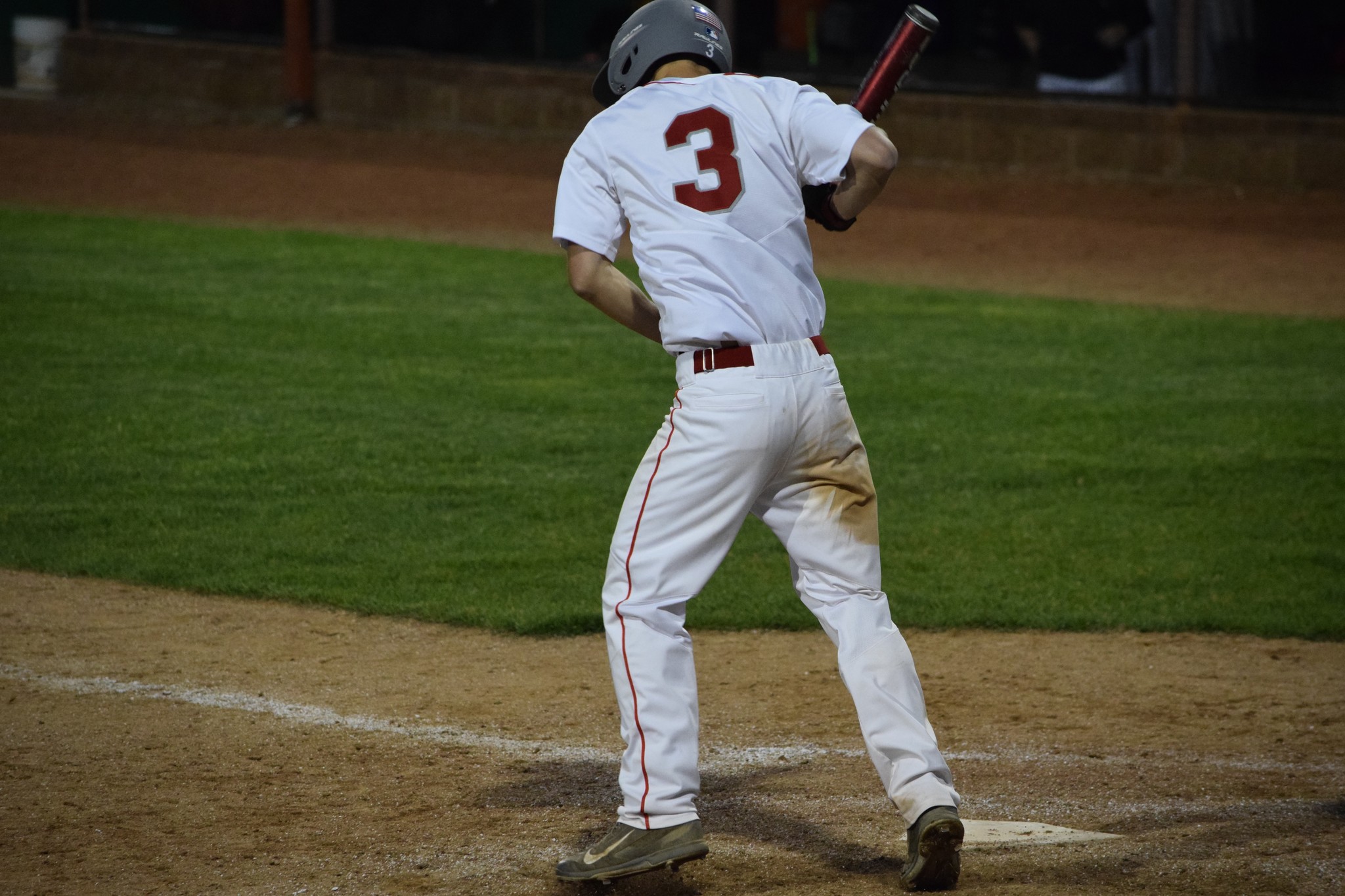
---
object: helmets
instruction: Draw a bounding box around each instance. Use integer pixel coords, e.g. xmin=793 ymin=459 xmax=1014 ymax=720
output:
xmin=593 ymin=0 xmax=731 ymax=103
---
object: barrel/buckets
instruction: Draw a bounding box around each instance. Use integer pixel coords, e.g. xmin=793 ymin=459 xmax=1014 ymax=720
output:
xmin=9 ymin=17 xmax=63 ymax=90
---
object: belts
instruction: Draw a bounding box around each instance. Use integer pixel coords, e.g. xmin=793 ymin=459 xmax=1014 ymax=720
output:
xmin=696 ymin=336 xmax=829 ymax=374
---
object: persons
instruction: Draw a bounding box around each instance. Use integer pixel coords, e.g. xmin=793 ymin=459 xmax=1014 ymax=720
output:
xmin=556 ymin=0 xmax=965 ymax=890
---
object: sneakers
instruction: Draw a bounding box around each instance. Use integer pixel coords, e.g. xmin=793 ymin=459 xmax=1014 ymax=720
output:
xmin=554 ymin=816 xmax=708 ymax=880
xmin=900 ymin=805 xmax=964 ymax=891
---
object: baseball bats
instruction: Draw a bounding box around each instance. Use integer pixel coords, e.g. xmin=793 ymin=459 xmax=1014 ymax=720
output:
xmin=850 ymin=4 xmax=939 ymax=125
